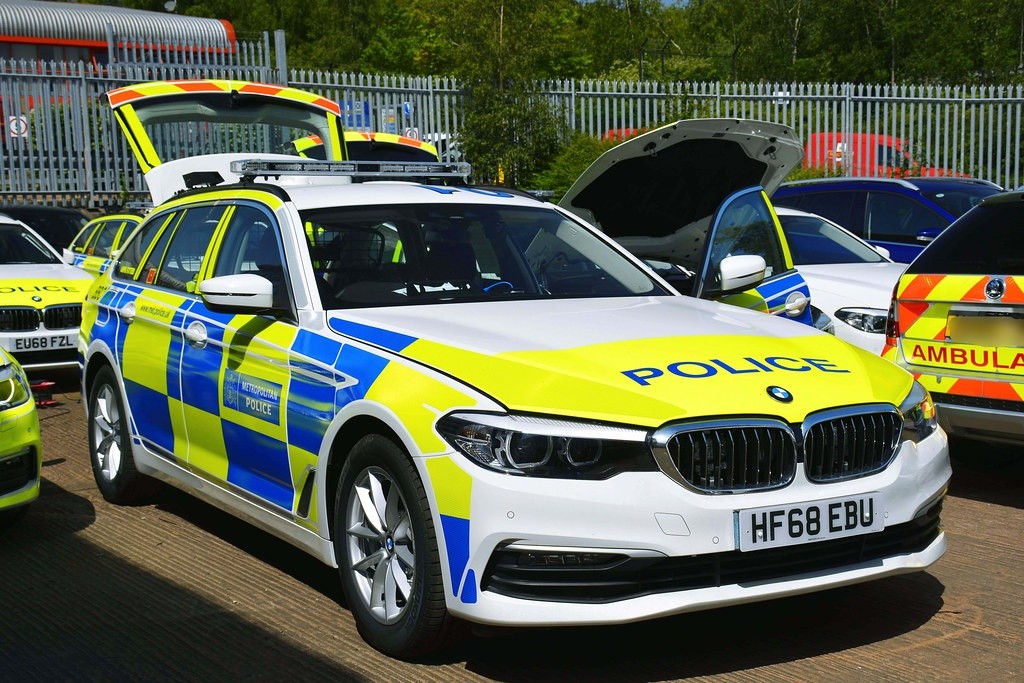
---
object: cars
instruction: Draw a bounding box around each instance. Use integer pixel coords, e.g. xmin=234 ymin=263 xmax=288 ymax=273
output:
xmin=317 ymin=116 xmax=805 ymax=306
xmin=423 ymin=133 xmax=466 ymax=165
xmin=0 ymin=343 xmax=44 ymax=516
xmin=773 ymin=208 xmax=908 ymax=363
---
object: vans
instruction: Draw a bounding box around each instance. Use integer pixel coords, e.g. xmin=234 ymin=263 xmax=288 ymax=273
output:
xmin=0 ymin=204 xmax=96 ymax=259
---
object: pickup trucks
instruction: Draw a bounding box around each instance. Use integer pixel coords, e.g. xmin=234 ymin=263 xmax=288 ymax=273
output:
xmin=802 ymin=132 xmax=963 ymax=179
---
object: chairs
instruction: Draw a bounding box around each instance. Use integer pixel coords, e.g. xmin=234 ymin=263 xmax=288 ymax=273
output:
xmin=429 ymin=240 xmax=483 ymax=285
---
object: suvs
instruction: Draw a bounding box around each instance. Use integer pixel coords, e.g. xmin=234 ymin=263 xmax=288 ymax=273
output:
xmin=63 ymin=212 xmax=149 ymax=278
xmin=880 ymin=190 xmax=1024 ymax=448
xmin=0 ymin=214 xmax=96 ymax=379
xmin=770 ymin=176 xmax=1009 ymax=264
xmin=77 ymin=80 xmax=952 ymax=663
